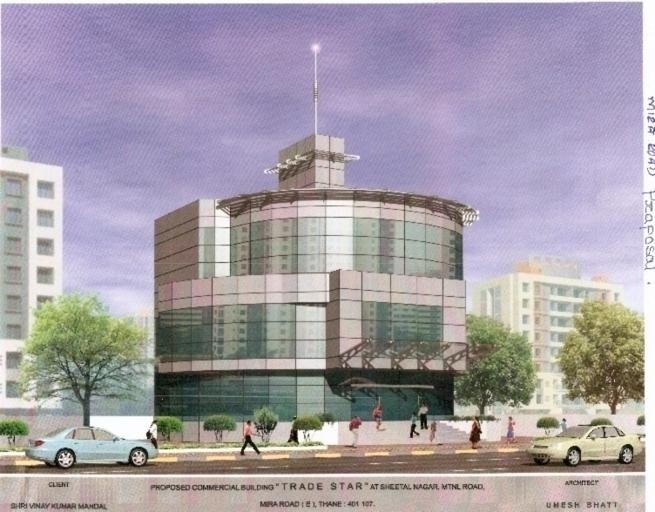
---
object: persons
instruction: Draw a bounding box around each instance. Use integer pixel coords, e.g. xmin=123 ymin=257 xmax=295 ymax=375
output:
xmin=148 ymin=420 xmax=158 ymax=450
xmin=287 ymin=416 xmax=299 ymax=445
xmin=349 ymin=415 xmax=361 ymax=448
xmin=417 ymin=402 xmax=428 ymax=430
xmin=560 ymin=418 xmax=567 ymax=432
xmin=469 ymin=417 xmax=482 ymax=449
xmin=507 ymin=416 xmax=516 ymax=447
xmin=409 ymin=412 xmax=419 ymax=438
xmin=240 ymin=419 xmax=262 ymax=456
xmin=373 ymin=404 xmax=383 ymax=430
